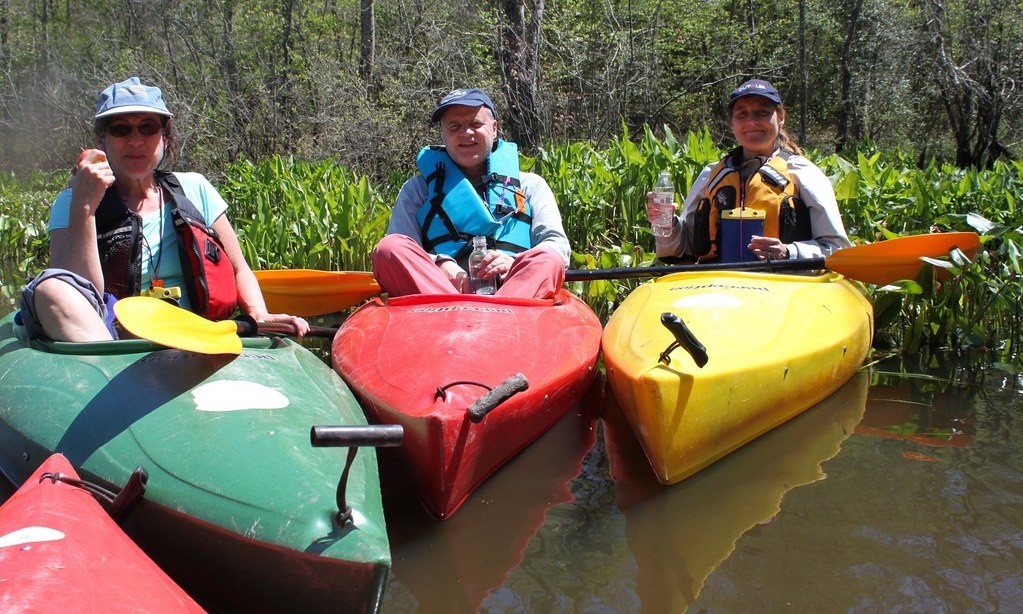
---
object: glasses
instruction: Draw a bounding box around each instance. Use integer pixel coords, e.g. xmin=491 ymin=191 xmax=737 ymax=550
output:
xmin=102 ymin=118 xmax=166 ymax=137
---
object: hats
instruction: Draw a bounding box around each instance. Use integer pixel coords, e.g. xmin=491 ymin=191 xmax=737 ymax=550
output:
xmin=94 ymin=76 xmax=173 ymax=118
xmin=431 ymin=86 xmax=497 ymax=122
xmin=726 ymin=78 xmax=781 ymax=107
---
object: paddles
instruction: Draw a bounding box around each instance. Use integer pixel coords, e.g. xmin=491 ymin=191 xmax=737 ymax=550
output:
xmin=249 ymin=231 xmax=979 ymax=317
xmin=113 ymin=296 xmax=340 ymax=356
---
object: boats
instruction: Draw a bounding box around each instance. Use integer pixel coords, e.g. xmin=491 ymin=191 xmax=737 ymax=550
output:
xmin=604 ymin=364 xmax=869 ymax=613
xmin=601 ymin=263 xmax=876 ymax=484
xmin=381 ymin=379 xmax=602 ymax=614
xmin=329 ymin=290 xmax=601 ymax=520
xmin=0 ymin=303 xmax=393 ymax=614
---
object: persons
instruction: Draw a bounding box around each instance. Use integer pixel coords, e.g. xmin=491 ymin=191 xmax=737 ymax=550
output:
xmin=22 ymin=77 xmax=311 ymax=343
xmin=371 ymin=88 xmax=574 ymax=299
xmin=645 ymin=77 xmax=851 ymax=272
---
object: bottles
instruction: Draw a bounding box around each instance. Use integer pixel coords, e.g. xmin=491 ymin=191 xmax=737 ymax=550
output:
xmin=651 ymin=171 xmax=674 ymax=238
xmin=469 ymin=236 xmax=498 ymax=295
